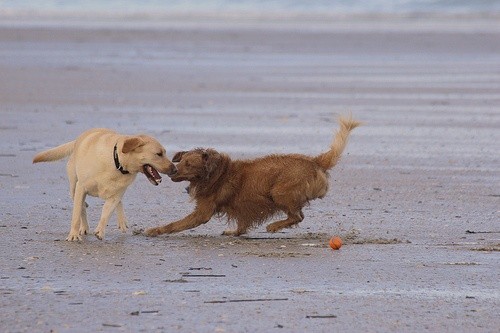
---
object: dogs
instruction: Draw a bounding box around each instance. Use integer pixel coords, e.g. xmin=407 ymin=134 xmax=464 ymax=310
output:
xmin=144 ymin=112 xmax=360 ymax=236
xmin=32 ymin=127 xmax=175 ymax=242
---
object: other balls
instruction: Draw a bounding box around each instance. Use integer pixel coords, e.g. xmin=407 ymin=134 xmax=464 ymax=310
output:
xmin=328 ymin=236 xmax=343 ymax=249
